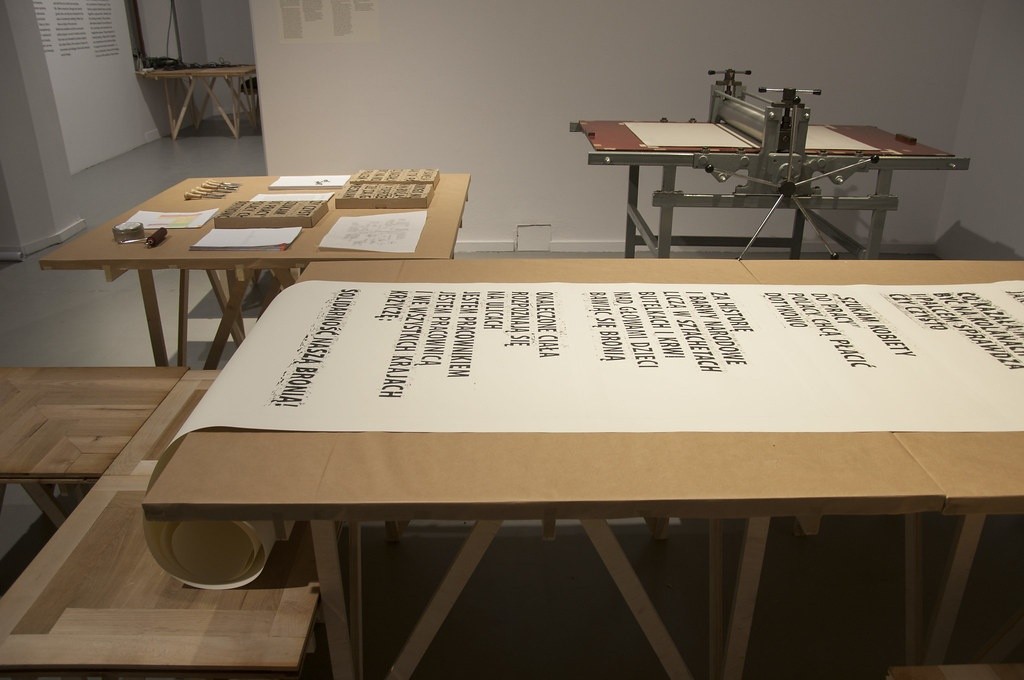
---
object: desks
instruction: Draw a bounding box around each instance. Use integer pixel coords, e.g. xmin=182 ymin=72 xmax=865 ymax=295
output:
xmin=37 ymin=172 xmax=473 ymax=369
xmin=142 ymin=253 xmax=1024 ymax=680
xmin=142 ymin=64 xmax=262 ymax=141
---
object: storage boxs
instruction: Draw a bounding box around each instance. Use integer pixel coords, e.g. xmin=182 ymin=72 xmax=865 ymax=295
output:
xmin=213 ymin=200 xmax=329 ymax=229
xmin=334 ymin=168 xmax=440 ymax=209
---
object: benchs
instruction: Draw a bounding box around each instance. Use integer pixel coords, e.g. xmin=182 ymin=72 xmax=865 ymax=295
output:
xmin=0 ymin=365 xmax=188 ymax=529
xmin=0 ymin=365 xmax=339 ymax=680
